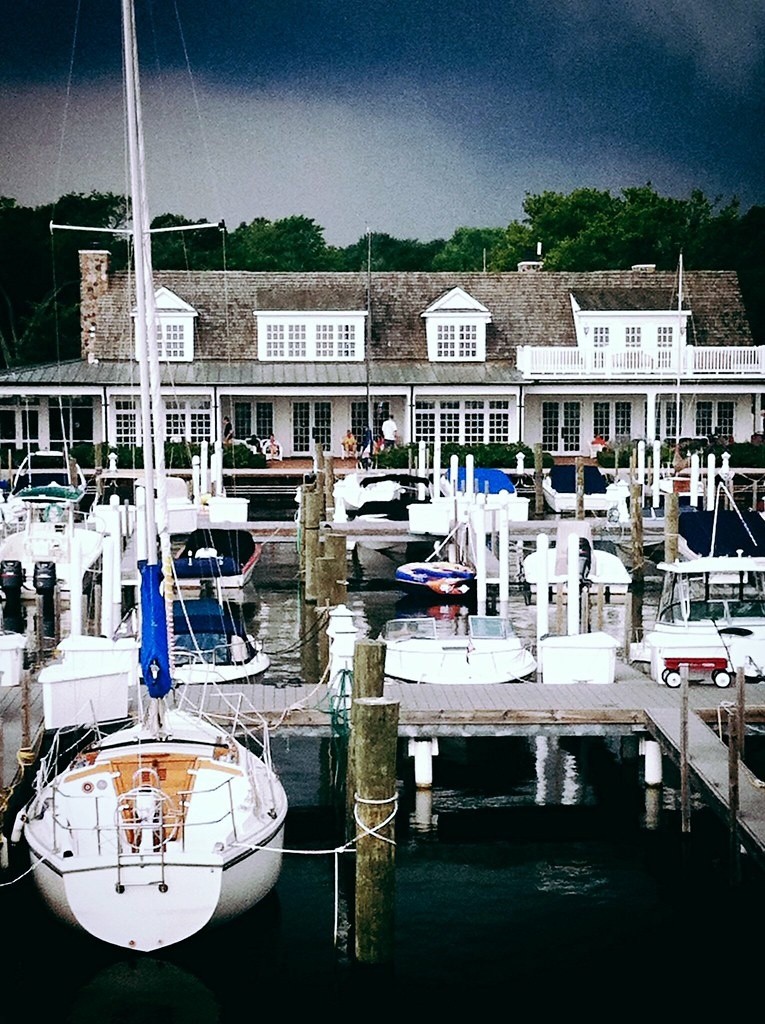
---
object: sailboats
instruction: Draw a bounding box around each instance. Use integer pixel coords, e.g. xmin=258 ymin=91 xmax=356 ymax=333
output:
xmin=0 ymin=4 xmax=289 ymax=951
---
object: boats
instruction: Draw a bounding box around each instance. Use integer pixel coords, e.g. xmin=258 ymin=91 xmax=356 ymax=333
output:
xmin=0 ymin=438 xmax=765 ymax=713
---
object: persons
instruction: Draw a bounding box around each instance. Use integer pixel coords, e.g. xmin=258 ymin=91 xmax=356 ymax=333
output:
xmin=591 ymin=435 xmax=616 ymax=462
xmin=672 ymin=434 xmax=763 ymax=492
xmin=342 ymin=415 xmax=397 ymax=456
xmin=222 ymin=417 xmax=278 ymax=456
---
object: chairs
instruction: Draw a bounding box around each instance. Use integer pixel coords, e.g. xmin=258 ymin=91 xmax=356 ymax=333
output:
xmin=341 ymin=435 xmax=359 ymax=460
